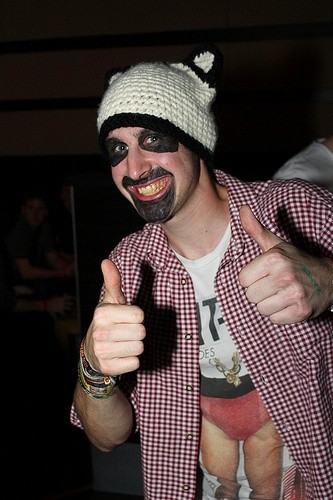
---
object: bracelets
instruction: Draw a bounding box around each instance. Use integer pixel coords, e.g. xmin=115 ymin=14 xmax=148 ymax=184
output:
xmin=77 ymin=336 xmax=122 ymax=399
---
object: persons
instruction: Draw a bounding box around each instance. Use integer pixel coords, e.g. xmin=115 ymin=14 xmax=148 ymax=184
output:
xmin=272 ymin=132 xmax=333 ymax=192
xmin=69 ymin=52 xmax=332 ymax=500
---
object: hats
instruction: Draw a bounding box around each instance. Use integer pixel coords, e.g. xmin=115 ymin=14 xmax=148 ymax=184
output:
xmin=90 ymin=50 xmax=219 ymax=154
